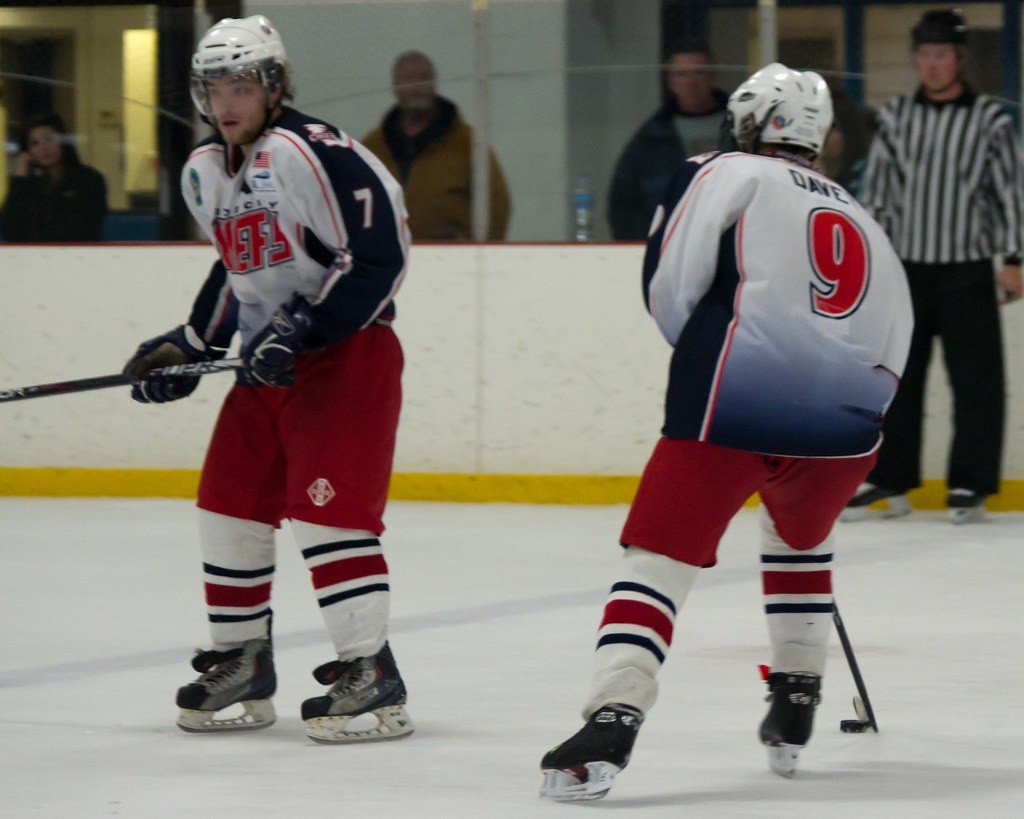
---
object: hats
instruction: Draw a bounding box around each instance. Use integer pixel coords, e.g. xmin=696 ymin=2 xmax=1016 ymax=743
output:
xmin=17 ymin=111 xmax=68 ymax=150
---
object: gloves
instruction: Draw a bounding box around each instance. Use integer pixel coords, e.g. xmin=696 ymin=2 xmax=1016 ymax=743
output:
xmin=244 ymin=289 xmax=326 ymax=392
xmin=123 ymin=325 xmax=210 ymax=403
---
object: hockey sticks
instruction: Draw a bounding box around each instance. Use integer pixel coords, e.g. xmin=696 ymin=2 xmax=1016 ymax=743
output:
xmin=830 ymin=597 xmax=882 ymax=734
xmin=1 ymin=358 xmax=247 ymax=405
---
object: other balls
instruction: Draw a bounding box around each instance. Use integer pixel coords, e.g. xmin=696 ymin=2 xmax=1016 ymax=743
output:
xmin=840 ymin=719 xmax=870 ymax=734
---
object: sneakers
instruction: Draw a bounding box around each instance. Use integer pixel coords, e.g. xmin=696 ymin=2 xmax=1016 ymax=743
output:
xmin=841 ymin=487 xmax=909 ymax=520
xmin=945 ymin=489 xmax=986 ymax=523
xmin=176 ymin=636 xmax=278 ymax=731
xmin=759 ymin=672 xmax=822 ymax=778
xmin=300 ymin=640 xmax=415 ymax=743
xmin=538 ymin=702 xmax=643 ymax=799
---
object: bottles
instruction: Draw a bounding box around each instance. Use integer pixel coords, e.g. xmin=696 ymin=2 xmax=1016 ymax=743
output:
xmin=572 ymin=171 xmax=595 ymax=243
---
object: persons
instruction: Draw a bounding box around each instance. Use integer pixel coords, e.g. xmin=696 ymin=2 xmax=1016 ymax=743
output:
xmin=0 ymin=113 xmax=106 ymax=245
xmin=854 ymin=7 xmax=1023 ymax=524
xmin=121 ymin=13 xmax=421 ymax=745
xmin=610 ymin=43 xmax=733 ymax=242
xmin=538 ymin=61 xmax=915 ymax=807
xmin=360 ymin=45 xmax=516 ymax=246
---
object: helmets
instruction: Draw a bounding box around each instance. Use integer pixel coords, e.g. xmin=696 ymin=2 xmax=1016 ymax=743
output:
xmin=725 ymin=57 xmax=836 ymax=157
xmin=194 ymin=13 xmax=296 ymax=122
xmin=911 ymin=6 xmax=971 ymax=47
